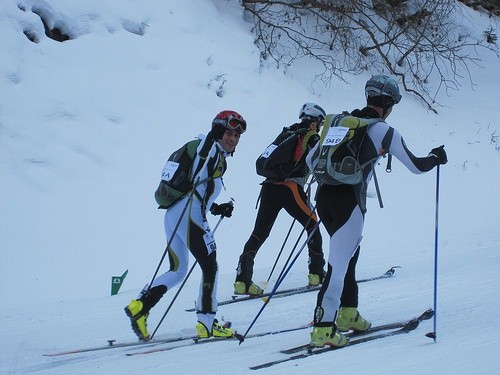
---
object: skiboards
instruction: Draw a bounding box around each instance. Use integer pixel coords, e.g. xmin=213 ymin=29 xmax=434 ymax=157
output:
xmin=184 ymin=260 xmax=404 ymax=314
xmin=247 ymin=307 xmax=434 ymax=371
xmin=41 ymin=320 xmax=314 ymax=365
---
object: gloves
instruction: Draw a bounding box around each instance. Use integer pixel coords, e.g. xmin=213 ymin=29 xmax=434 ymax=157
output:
xmin=211 ymin=122 xmax=225 ymax=141
xmin=215 ymin=203 xmax=233 ymax=217
xmin=430 ymin=144 xmax=447 ymax=164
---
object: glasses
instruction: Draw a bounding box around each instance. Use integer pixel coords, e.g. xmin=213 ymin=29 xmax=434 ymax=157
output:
xmin=228 ymin=118 xmax=247 ymax=131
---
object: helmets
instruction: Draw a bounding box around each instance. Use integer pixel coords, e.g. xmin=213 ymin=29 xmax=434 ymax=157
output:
xmin=365 ymin=74 xmax=401 ymax=103
xmin=212 ymin=109 xmax=245 ymax=134
xmin=299 ymin=102 xmax=326 ymax=120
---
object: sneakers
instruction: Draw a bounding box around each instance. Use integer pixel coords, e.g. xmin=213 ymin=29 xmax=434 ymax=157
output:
xmin=234 ymin=280 xmax=263 ymax=295
xmin=311 ymin=325 xmax=346 ymax=348
xmin=336 ymin=310 xmax=371 ymax=332
xmin=125 ymin=299 xmax=148 ymax=339
xmin=308 ymin=270 xmax=328 ymax=286
xmin=196 ymin=319 xmax=234 ymax=338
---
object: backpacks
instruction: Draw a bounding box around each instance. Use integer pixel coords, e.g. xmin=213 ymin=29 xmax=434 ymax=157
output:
xmin=155 ymin=139 xmax=227 ymax=208
xmin=256 ymin=126 xmax=313 ymax=179
xmin=312 ymin=113 xmax=389 ymax=184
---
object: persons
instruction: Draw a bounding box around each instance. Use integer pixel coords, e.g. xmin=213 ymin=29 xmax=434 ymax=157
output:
xmin=233 ymin=104 xmax=328 ymax=296
xmin=305 ymin=76 xmax=447 ymax=348
xmin=123 ymin=110 xmax=246 ymax=341
